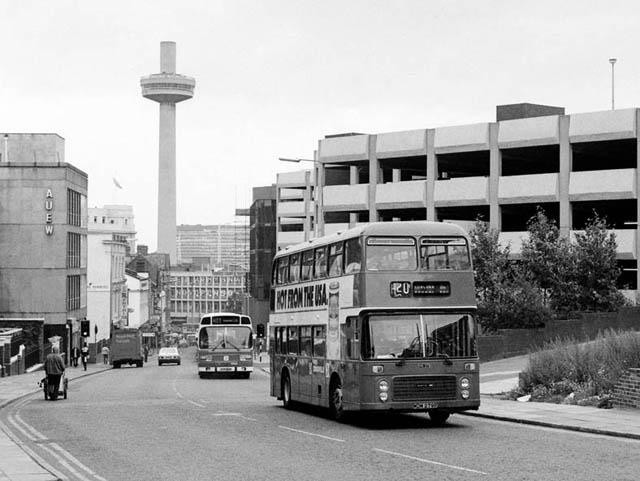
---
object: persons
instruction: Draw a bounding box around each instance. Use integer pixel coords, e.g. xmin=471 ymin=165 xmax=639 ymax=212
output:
xmin=102 ymin=344 xmax=109 ymax=365
xmin=80 ymin=342 xmax=89 ymax=372
xmin=45 ymin=347 xmax=65 ymax=401
xmin=70 ymin=344 xmax=80 ymax=367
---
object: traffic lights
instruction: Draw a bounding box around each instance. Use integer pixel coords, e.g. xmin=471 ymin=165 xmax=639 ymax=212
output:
xmin=80 ymin=320 xmax=89 ymax=338
xmin=256 ymin=324 xmax=264 ymax=339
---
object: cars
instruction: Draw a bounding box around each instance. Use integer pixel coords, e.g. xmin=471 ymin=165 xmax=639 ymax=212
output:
xmin=178 ymin=334 xmax=195 ymax=347
xmin=159 ymin=347 xmax=182 ymax=365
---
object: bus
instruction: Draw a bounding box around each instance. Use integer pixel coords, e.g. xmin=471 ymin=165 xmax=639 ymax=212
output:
xmin=108 ymin=328 xmax=144 ymax=370
xmin=265 ymin=222 xmax=480 ymax=422
xmin=196 ymin=312 xmax=254 ymax=377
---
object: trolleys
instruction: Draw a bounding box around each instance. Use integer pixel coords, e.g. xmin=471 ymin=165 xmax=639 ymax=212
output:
xmin=42 ymin=374 xmax=68 ymax=401
